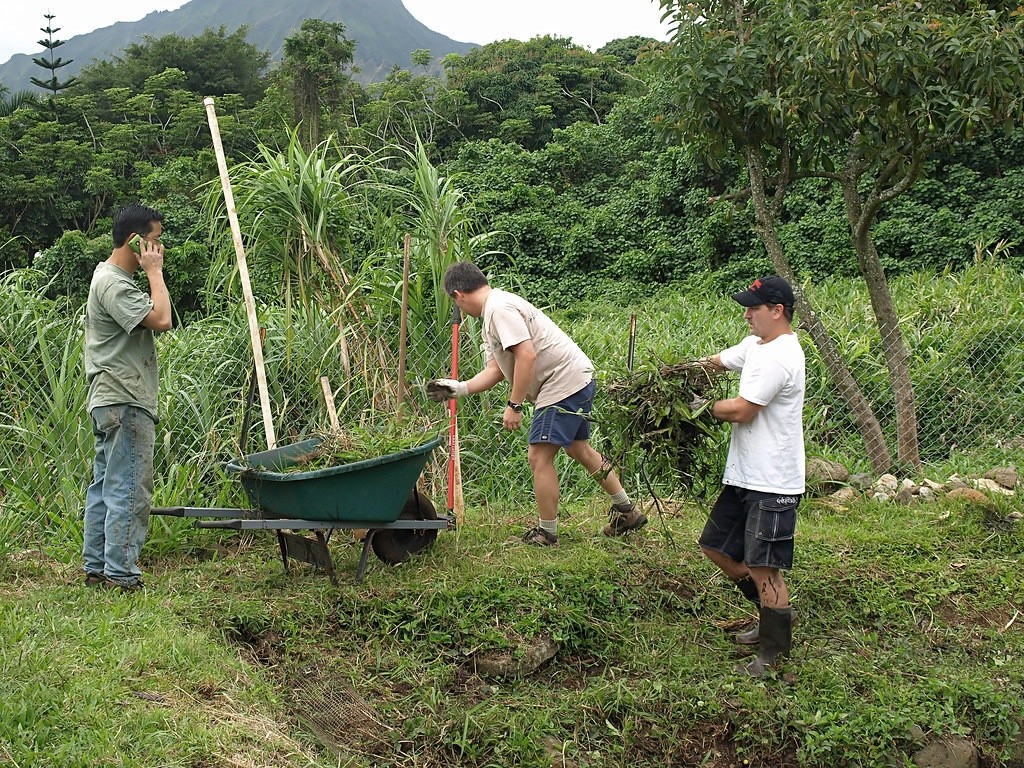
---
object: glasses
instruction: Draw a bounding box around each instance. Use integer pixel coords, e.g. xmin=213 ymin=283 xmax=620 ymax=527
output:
xmin=138 ymin=234 xmax=164 ymax=246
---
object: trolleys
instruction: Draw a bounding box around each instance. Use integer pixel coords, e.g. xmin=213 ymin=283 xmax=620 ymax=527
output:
xmin=148 ymin=413 xmax=457 ymax=589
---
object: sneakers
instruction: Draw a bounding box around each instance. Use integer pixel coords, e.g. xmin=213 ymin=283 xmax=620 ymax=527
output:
xmin=507 ymin=527 xmax=560 ymax=548
xmin=603 ymin=501 xmax=648 ymax=537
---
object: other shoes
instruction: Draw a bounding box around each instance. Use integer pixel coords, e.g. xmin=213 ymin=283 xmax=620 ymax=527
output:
xmin=104 ymin=580 xmax=144 ymax=594
xmin=89 ymin=576 xmax=101 ymax=586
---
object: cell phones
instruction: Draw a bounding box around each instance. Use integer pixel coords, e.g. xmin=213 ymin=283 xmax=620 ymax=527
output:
xmin=127 ymin=234 xmax=147 ymax=255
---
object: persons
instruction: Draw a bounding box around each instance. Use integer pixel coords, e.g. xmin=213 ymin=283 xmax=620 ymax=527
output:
xmin=426 ymin=260 xmax=647 ymax=546
xmin=82 ymin=204 xmax=172 ymax=595
xmin=689 ymin=275 xmax=807 ymax=677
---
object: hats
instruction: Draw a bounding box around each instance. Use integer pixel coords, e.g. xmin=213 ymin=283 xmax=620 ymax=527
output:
xmin=730 ymin=275 xmax=795 ymax=308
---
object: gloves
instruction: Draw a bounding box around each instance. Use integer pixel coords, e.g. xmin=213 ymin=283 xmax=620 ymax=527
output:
xmin=426 ymin=379 xmax=469 ymax=402
xmin=688 ymin=391 xmax=709 ymax=415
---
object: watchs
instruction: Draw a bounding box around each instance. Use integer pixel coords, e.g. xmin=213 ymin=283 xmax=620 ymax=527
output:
xmin=508 ymin=401 xmax=524 ymax=414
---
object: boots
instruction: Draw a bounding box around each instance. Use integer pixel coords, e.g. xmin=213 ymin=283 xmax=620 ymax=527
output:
xmin=735 ymin=607 xmax=793 ymax=678
xmin=735 ymin=574 xmax=764 ymax=645
